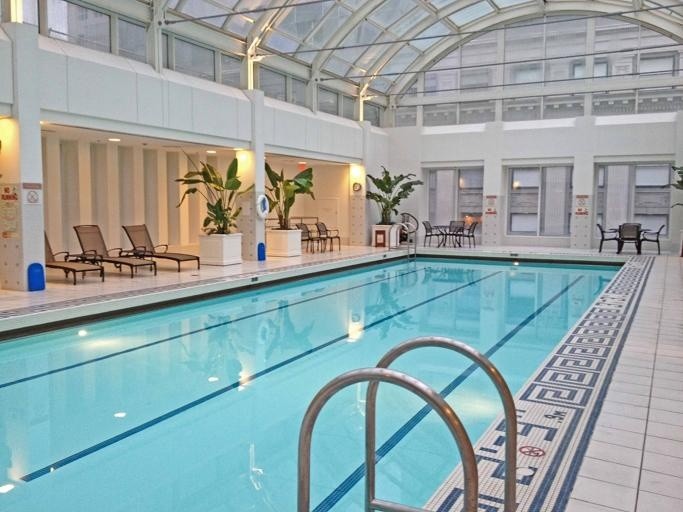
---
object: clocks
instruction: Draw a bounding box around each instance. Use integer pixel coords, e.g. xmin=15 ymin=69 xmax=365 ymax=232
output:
xmin=351 ymin=182 xmax=361 ymax=192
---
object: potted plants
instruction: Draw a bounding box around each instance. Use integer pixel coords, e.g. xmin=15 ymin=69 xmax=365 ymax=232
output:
xmin=365 ymin=164 xmax=425 ymax=251
xmin=260 ymin=159 xmax=317 ymax=258
xmin=169 ymin=143 xmax=256 ymax=267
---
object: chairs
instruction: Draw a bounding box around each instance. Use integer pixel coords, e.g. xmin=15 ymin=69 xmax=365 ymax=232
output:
xmin=71 ymin=224 xmax=156 ymax=278
xmin=42 ymin=230 xmax=104 ymax=286
xmin=595 ymin=222 xmax=666 ymax=256
xmin=293 ymin=221 xmax=341 ymax=253
xmin=120 ymin=223 xmax=201 ymax=273
xmin=420 ymin=218 xmax=478 ymax=249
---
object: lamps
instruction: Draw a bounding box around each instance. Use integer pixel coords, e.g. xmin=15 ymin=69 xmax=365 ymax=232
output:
xmin=362 ymin=94 xmax=376 ymax=102
xmin=249 ymin=52 xmax=266 ymax=64
